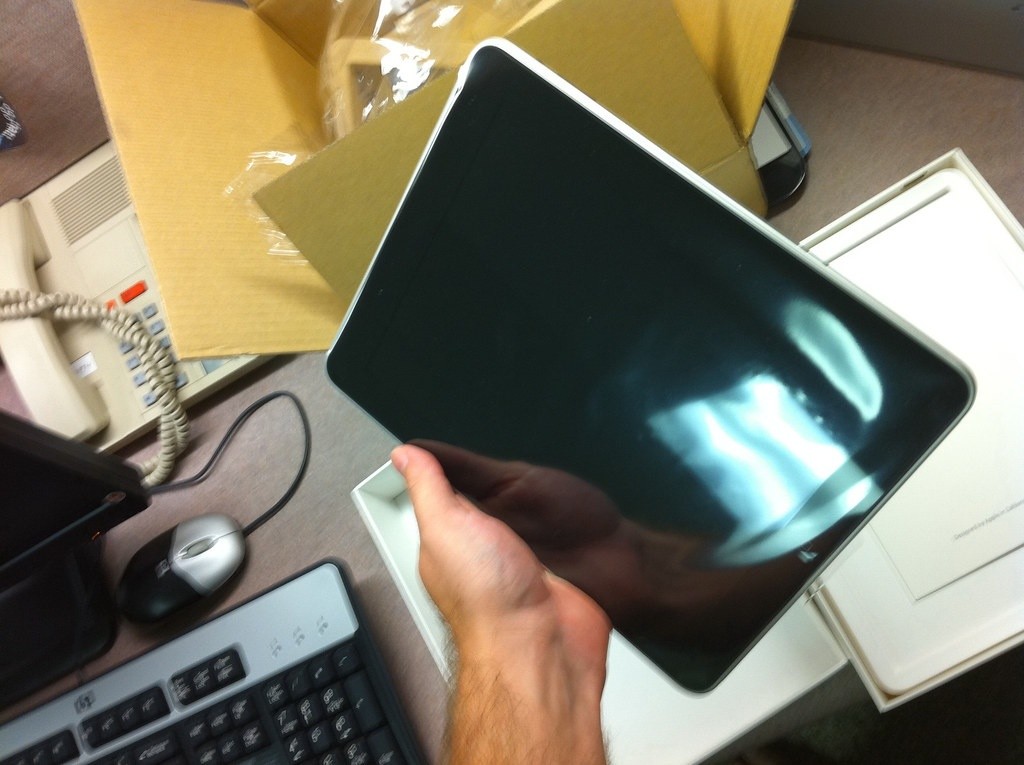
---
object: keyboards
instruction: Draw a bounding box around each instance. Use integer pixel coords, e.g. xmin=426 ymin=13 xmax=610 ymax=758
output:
xmin=0 ymin=553 xmax=428 ymax=765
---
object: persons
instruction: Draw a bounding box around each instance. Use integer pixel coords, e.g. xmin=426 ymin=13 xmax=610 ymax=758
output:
xmin=387 ymin=441 xmax=613 ymax=764
xmin=404 ymin=437 xmax=845 ymax=623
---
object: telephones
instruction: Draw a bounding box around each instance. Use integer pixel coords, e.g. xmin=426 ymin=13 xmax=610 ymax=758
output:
xmin=0 ymin=137 xmax=281 ymax=455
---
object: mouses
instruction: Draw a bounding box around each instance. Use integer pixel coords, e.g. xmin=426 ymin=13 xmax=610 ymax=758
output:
xmin=116 ymin=511 xmax=246 ymax=625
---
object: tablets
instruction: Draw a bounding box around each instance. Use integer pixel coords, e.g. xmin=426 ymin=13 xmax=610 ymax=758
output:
xmin=328 ymin=38 xmax=977 ymax=696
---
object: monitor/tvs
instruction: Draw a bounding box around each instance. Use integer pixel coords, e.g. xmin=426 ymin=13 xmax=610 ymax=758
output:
xmin=0 ymin=409 xmax=153 ymax=713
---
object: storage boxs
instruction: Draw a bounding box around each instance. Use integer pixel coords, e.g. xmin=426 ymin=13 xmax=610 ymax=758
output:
xmin=71 ymin=0 xmax=796 ymax=363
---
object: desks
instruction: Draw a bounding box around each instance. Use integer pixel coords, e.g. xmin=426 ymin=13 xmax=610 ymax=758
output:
xmin=0 ymin=0 xmax=1024 ymax=765
